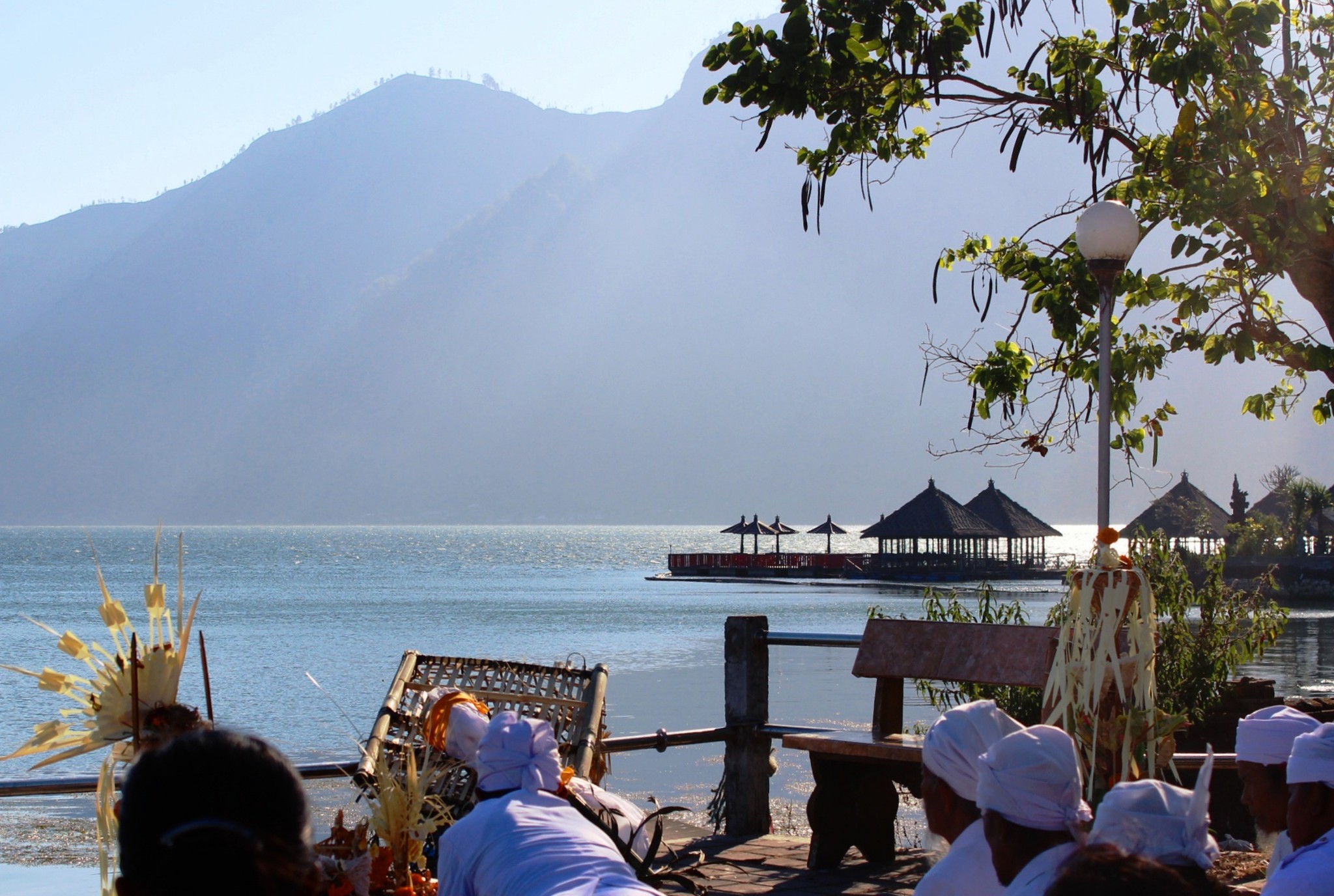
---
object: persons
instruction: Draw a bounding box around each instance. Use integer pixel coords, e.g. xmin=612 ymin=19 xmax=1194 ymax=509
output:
xmin=107 ymin=700 xmax=326 ymax=895
xmin=435 ymin=710 xmax=669 ymax=896
xmin=905 ymin=699 xmax=1334 ymax=896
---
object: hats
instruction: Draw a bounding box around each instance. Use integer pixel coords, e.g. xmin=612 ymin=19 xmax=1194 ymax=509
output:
xmin=1085 ymin=743 xmax=1219 ymax=868
xmin=976 ymin=724 xmax=1093 ymax=844
xmin=1234 ymin=705 xmax=1322 ymax=765
xmin=921 ymin=699 xmax=1026 ymax=801
xmin=473 ymin=710 xmax=562 ymax=796
xmin=1286 ymin=722 xmax=1334 ymax=787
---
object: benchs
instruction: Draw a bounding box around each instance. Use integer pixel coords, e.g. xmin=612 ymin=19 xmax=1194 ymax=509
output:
xmin=783 ymin=618 xmax=1161 ymax=877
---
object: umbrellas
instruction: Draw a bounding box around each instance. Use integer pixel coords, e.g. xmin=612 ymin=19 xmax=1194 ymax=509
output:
xmin=806 ymin=514 xmax=847 ymax=554
xmin=720 ymin=514 xmax=800 ymax=556
